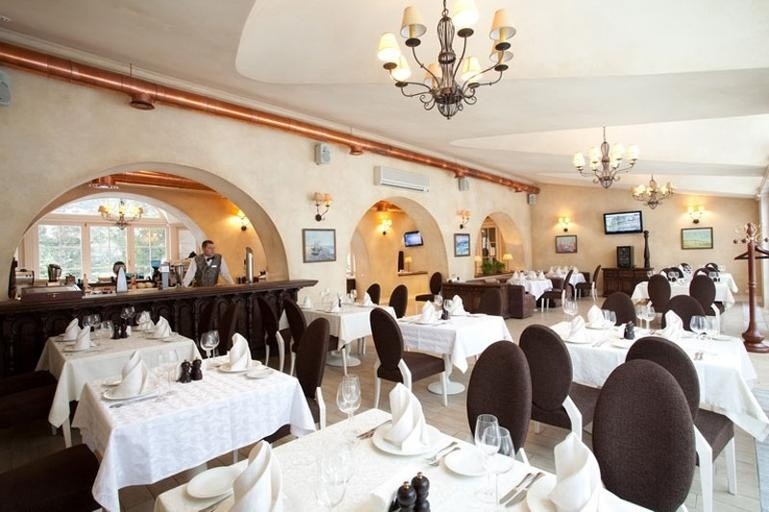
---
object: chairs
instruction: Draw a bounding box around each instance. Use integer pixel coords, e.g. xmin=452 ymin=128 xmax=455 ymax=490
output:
xmin=690 ymin=276 xmax=725 ymax=331
xmin=648 ymin=274 xmax=672 ymax=314
xmin=590 ymin=358 xmax=692 ymax=512
xmin=467 ymin=341 xmax=533 ymax=456
xmin=622 ymin=341 xmax=737 ymax=496
xmin=366 ymin=284 xmax=381 ymax=307
xmin=215 ymin=299 xmax=242 ymax=352
xmin=660 ymin=295 xmax=706 ymax=332
xmin=510 ymin=325 xmax=590 ymax=429
xmin=414 ymin=272 xmax=442 ymax=316
xmin=599 ymin=291 xmax=637 ymax=325
xmin=388 ymin=284 xmax=408 ymax=318
xmin=281 ymin=298 xmax=349 ymax=381
xmin=365 ymin=310 xmax=450 ymax=413
xmin=478 ymin=289 xmax=502 ymax=318
xmin=255 ymin=298 xmax=292 ymax=369
xmin=445 ymin=262 xmax=737 ymax=310
xmin=265 ymin=318 xmax=331 ymax=442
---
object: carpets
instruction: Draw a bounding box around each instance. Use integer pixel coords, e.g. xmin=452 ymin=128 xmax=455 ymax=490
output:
xmin=752 ymin=388 xmax=769 ymax=512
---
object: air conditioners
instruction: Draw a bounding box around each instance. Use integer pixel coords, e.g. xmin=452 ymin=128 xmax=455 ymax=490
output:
xmin=373 ymin=165 xmax=430 ymax=192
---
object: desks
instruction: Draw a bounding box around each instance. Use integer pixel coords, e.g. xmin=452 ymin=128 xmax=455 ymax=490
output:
xmin=542 ymin=318 xmax=737 ymax=408
xmin=299 ymin=298 xmax=396 ymax=377
xmin=80 ymin=354 xmax=313 ymax=495
xmin=385 ymin=309 xmax=515 ymax=408
xmin=162 ymin=409 xmax=647 ymax=509
xmin=49 ymin=321 xmax=197 ymax=435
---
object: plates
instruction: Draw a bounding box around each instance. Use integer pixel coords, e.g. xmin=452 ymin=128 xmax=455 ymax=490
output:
xmin=413 ymin=319 xmax=435 ymax=325
xmin=102 ymin=374 xmax=169 ymax=400
xmin=372 ymin=422 xmax=498 ymax=478
xmin=187 ymin=467 xmax=240 ymax=498
xmin=526 ymin=475 xmax=557 ymax=512
xmin=219 ymin=360 xmax=275 ymax=378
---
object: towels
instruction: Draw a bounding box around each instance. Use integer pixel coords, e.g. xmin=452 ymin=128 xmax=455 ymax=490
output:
xmin=63 ymin=318 xmax=81 ymax=341
xmin=149 ymin=316 xmax=174 ymax=339
xmin=136 ymin=310 xmax=154 ymax=331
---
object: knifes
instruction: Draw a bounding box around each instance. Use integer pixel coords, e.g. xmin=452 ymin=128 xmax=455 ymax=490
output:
xmin=500 ymin=472 xmax=545 ymax=507
xmin=356 ymin=419 xmax=392 ymax=440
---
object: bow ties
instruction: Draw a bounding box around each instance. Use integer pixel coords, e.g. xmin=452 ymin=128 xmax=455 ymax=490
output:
xmin=207 ymin=256 xmax=214 ymax=261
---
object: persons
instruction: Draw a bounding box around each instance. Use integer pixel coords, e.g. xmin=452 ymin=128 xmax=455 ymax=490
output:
xmin=181 ymin=239 xmax=237 ymax=287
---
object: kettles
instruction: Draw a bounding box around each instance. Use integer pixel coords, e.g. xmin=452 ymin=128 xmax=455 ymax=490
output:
xmin=48 ymin=264 xmax=62 ymax=281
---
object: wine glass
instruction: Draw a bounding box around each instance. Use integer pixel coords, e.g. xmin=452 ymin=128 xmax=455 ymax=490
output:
xmin=337 ymin=375 xmax=361 ymax=426
xmin=121 ymin=305 xmax=147 ymax=327
xmin=558 ymin=299 xmax=712 ymax=348
xmin=82 ymin=312 xmax=115 ymax=348
xmin=474 ymin=414 xmax=515 ymax=512
xmin=200 ymin=329 xmax=220 ymax=361
xmin=709 ymin=272 xmax=720 ymax=282
xmin=668 ymin=271 xmax=679 ymax=284
xmin=431 ymin=293 xmax=452 ymax=322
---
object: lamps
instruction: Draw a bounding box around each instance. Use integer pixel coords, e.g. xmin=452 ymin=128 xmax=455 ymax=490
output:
xmin=228 ymin=210 xmax=250 ymax=231
xmin=687 ymin=206 xmax=705 ymax=225
xmin=558 ymin=217 xmax=571 ymax=233
xmin=503 ymin=253 xmax=513 ymax=273
xmin=572 ymin=127 xmax=639 ymax=189
xmin=97 ymin=198 xmax=145 ymax=231
xmin=631 ymin=175 xmax=677 ymax=211
xmin=311 ymin=192 xmax=333 ymax=222
xmin=473 ymin=255 xmax=482 ymax=278
xmin=456 ymin=208 xmax=472 ymax=229
xmin=376 ymin=0 xmax=519 ymax=123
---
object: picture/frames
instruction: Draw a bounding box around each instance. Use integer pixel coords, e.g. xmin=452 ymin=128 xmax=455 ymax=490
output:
xmin=454 ymin=233 xmax=470 ymax=257
xmin=680 ymin=227 xmax=714 ymax=250
xmin=554 ymin=234 xmax=578 ymax=254
xmin=302 ymin=228 xmax=337 ymax=263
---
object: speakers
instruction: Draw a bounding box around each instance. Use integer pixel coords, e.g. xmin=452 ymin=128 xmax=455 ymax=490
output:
xmin=528 ymin=193 xmax=537 ymax=205
xmin=316 ymin=143 xmax=331 ymax=163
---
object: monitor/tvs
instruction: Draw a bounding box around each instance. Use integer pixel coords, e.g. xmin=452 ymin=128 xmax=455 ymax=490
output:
xmin=404 ymin=230 xmax=423 ymax=247
xmin=604 ymin=210 xmax=643 ymax=234
